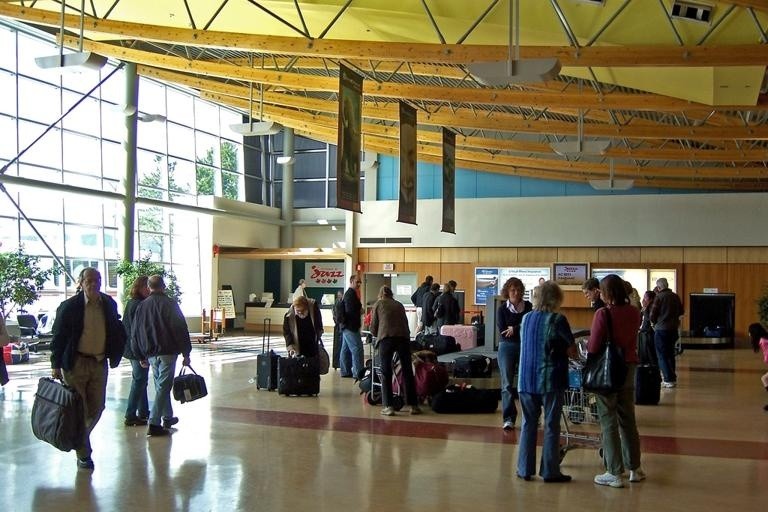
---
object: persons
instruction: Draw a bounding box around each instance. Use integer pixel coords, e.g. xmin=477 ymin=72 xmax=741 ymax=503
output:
xmin=339 ymin=275 xmax=364 ymax=379
xmin=371 ymin=284 xmax=423 ymax=414
xmin=51 ymin=269 xmax=126 ymax=468
xmin=497 ymin=275 xmax=684 ymax=488
xmin=283 ymin=297 xmax=323 ymax=363
xmin=131 ymin=276 xmax=192 ymax=436
xmin=0 ymin=313 xmax=10 ymax=383
xmin=411 ymin=276 xmax=459 ymax=334
xmin=292 ymin=280 xmax=313 ymax=303
xmin=121 ymin=276 xmax=152 ymax=425
xmin=748 ymin=323 xmax=768 ymax=410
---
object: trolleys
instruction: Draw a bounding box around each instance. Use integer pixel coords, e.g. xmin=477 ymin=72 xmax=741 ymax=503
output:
xmin=365 ymin=335 xmax=404 ymax=412
xmin=559 ymin=362 xmax=604 ymax=466
xmin=17 ymin=324 xmax=54 ymax=353
xmin=188 ymin=305 xmax=226 ymax=345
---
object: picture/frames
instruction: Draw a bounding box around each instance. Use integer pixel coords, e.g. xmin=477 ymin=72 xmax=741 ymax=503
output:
xmin=553 ymin=263 xmax=588 ymax=285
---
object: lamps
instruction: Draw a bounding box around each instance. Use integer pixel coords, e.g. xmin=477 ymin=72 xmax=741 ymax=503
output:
xmin=113 ymin=101 xmax=138 ymax=118
xmin=277 ymin=156 xmax=295 ymax=166
xmin=137 ymin=110 xmax=167 ymax=125
xmin=229 ymin=52 xmax=283 ymax=139
xmin=547 ymin=77 xmax=611 ymax=158
xmin=35 ymin=2 xmax=108 ymax=71
xmin=467 ymin=0 xmax=562 ymax=91
xmin=587 ymin=157 xmax=633 ymax=190
xmin=360 ymin=159 xmax=381 ymax=172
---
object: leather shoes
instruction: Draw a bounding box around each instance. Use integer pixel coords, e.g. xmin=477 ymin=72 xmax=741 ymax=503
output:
xmin=125 ymin=411 xmax=178 ymax=435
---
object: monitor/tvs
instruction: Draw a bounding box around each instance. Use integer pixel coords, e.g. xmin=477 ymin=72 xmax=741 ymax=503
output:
xmin=261 ymin=292 xmax=273 ymax=301
xmin=322 ymin=294 xmax=335 ymax=305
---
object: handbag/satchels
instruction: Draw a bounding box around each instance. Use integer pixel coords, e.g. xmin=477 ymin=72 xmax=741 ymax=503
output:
xmin=434 ymin=297 xmax=444 ymax=318
xmin=31 ymin=378 xmax=84 ymax=452
xmin=581 ymin=343 xmax=627 ymax=390
xmin=317 ymin=344 xmax=329 ymax=375
xmin=173 ymin=364 xmax=207 ymax=404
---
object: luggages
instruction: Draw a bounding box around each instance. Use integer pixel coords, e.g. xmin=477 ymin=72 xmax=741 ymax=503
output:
xmin=257 ymin=318 xmax=320 ymax=396
xmin=635 ymin=330 xmax=661 ymax=404
xmin=359 ymin=324 xmax=498 ymax=413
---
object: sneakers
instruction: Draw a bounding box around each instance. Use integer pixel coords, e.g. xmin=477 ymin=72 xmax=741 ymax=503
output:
xmin=503 ymin=421 xmax=515 ymax=429
xmin=516 ymin=467 xmax=646 ymax=487
xmin=662 ymin=379 xmax=676 ymax=388
xmin=77 ymin=457 xmax=93 ymax=468
xmin=380 ymin=407 xmax=423 ymax=416
